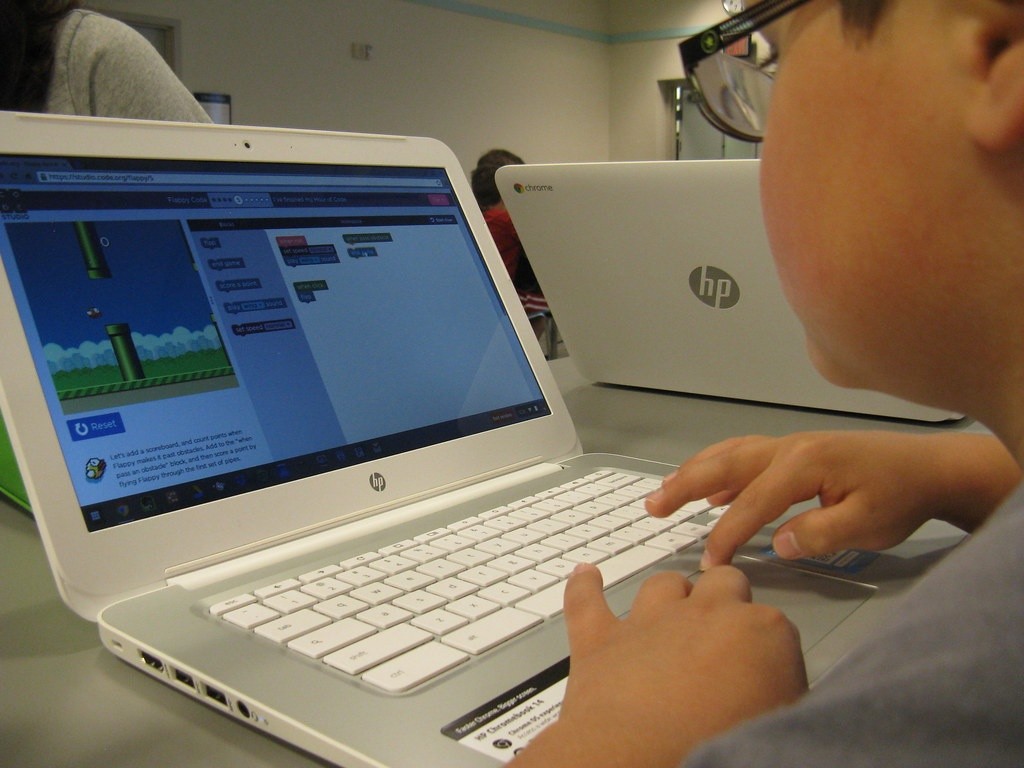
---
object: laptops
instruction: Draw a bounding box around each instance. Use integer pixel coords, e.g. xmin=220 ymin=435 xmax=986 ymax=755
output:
xmin=1 ymin=110 xmax=955 ymax=768
xmin=492 ymin=158 xmax=969 ymax=429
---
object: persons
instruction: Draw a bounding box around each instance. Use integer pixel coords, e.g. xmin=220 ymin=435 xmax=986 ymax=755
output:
xmin=471 ymin=150 xmax=553 ymax=341
xmin=501 ymin=1 xmax=1024 ymax=768
xmin=0 ymin=1 xmax=214 ymax=124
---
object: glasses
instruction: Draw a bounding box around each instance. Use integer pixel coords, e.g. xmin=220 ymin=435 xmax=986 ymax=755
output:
xmin=678 ymin=0 xmax=813 ymax=143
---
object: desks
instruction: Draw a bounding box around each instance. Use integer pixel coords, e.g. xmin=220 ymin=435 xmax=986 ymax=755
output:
xmin=0 ymin=356 xmax=1008 ymax=768
